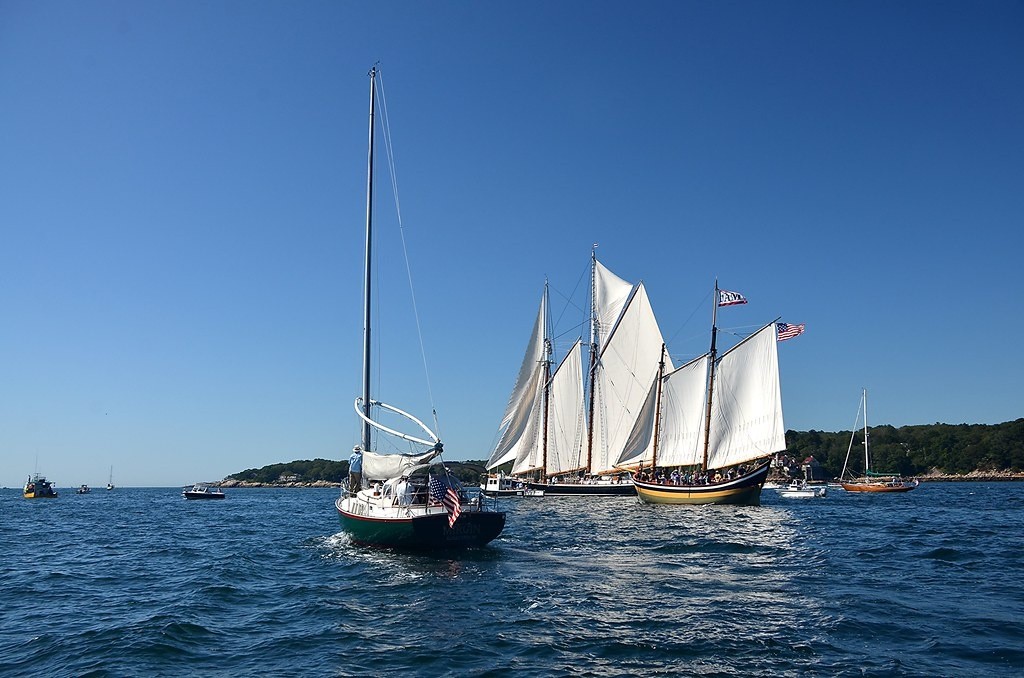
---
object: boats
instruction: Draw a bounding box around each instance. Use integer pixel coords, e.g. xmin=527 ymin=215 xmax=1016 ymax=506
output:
xmin=834 ymin=387 xmax=916 ymax=493
xmin=780 ymin=488 xmax=826 ymax=500
xmin=181 ymin=482 xmax=225 ymax=500
xmin=762 ymin=481 xmax=785 ymax=490
xmin=78 ymin=485 xmax=92 ymax=494
xmin=107 ymin=465 xmax=115 ymax=491
xmin=23 ymin=472 xmax=57 ymax=499
xmin=774 ymin=479 xmax=826 ymax=496
xmin=522 ymin=489 xmax=544 ymax=497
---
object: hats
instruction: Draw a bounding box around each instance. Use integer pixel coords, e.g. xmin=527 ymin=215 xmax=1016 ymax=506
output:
xmin=399 ymin=475 xmax=410 ymax=479
xmin=716 ymin=471 xmax=719 ymax=472
xmin=352 ymin=445 xmax=362 ymax=452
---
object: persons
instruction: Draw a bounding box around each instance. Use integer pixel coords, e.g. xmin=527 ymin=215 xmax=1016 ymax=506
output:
xmin=396 ymin=474 xmax=419 ymax=505
xmin=348 ymin=445 xmax=362 ymax=498
xmin=637 ymin=461 xmax=760 ymax=486
xmin=895 ymin=479 xmax=915 ymax=488
xmin=545 ymin=475 xmax=622 ymax=484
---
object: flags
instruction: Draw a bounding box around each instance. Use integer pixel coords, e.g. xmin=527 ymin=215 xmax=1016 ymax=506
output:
xmin=776 ymin=323 xmax=804 ymax=341
xmin=429 ymin=475 xmax=462 ymax=528
xmin=718 ymin=289 xmax=748 ymax=307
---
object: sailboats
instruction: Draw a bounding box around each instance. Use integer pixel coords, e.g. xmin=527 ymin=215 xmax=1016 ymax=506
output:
xmin=612 ymin=276 xmax=787 ymax=506
xmin=335 ymin=62 xmax=509 ymax=551
xmin=478 ymin=280 xmax=550 ymax=496
xmin=511 ymin=244 xmax=675 ymax=498
